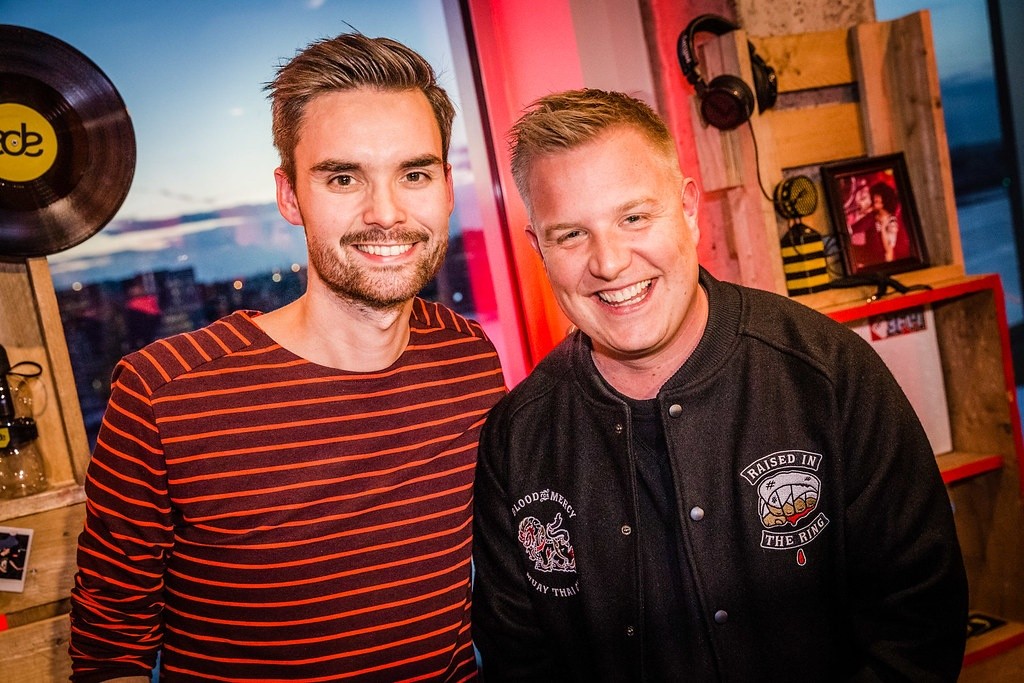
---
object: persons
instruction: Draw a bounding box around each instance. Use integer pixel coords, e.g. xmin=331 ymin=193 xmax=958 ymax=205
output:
xmin=848 ymin=181 xmax=899 ymax=267
xmin=65 ymin=19 xmax=511 ymax=683
xmin=470 ymin=88 xmax=973 ymax=683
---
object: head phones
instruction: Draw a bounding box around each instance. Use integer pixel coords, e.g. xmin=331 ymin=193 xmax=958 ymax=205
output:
xmin=676 ymin=14 xmax=776 ymax=130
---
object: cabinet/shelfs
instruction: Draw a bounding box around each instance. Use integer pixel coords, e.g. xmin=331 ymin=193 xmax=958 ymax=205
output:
xmin=740 ymin=272 xmax=1024 ymax=683
xmin=0 ymin=254 xmax=91 ymax=683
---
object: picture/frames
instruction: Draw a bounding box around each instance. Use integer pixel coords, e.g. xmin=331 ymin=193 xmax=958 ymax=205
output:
xmin=819 ymin=149 xmax=932 ymax=283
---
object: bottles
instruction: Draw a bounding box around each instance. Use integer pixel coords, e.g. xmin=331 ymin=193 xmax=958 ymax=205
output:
xmin=0 ymin=345 xmax=52 ymax=499
